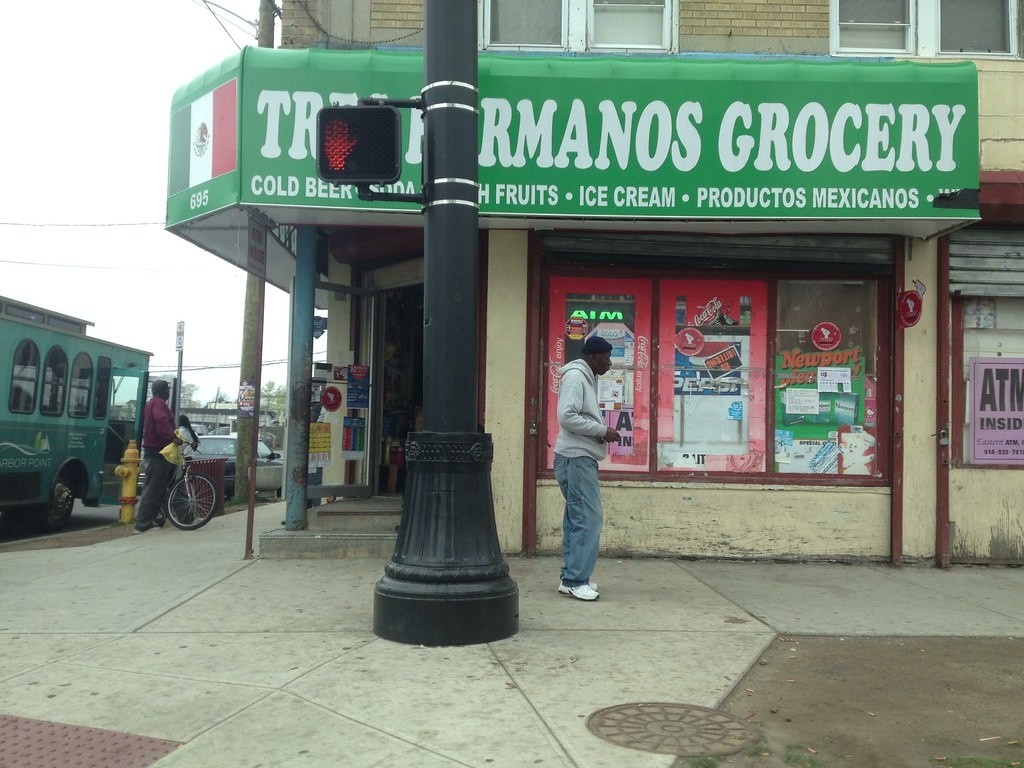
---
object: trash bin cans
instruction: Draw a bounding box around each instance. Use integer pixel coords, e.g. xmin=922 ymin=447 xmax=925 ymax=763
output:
xmin=184 ymin=458 xmax=227 ymax=518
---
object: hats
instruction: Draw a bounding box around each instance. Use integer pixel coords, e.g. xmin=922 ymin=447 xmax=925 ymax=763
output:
xmin=153 ymin=379 xmax=171 ymax=391
xmin=582 ymin=336 xmax=613 ymax=353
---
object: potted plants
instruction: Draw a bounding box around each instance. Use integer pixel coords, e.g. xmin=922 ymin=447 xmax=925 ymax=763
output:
xmin=247 ymin=461 xmax=284 ymax=501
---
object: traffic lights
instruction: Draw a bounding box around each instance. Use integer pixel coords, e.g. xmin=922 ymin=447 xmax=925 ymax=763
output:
xmin=315 ymin=105 xmax=406 ymax=183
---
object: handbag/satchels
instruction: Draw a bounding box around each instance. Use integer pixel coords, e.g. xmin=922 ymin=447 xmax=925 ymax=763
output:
xmin=159 ymin=428 xmax=184 ymax=466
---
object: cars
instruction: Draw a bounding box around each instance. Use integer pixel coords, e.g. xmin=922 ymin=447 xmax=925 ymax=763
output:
xmin=190 ymin=422 xmax=230 ymax=435
xmin=131 ymin=434 xmax=284 ymax=503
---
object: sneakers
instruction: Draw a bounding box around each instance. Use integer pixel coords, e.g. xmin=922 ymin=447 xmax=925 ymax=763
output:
xmin=133 ymin=517 xmax=164 ymax=534
xmin=559 ymin=580 xmax=599 ymax=600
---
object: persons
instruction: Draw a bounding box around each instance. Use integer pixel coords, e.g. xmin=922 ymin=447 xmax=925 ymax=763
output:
xmin=178 ymin=414 xmax=199 ymax=460
xmin=130 ymin=379 xmax=182 ymax=534
xmin=552 ymin=335 xmax=622 ymax=601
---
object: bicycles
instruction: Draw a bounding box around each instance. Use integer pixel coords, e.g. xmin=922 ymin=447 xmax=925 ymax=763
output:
xmin=147 ymin=437 xmax=220 ymax=531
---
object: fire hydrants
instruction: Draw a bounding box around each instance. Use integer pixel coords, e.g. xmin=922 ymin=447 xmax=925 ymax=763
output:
xmin=115 ymin=437 xmax=141 ymax=523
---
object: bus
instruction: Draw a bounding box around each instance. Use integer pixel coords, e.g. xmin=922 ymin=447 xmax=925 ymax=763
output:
xmin=0 ymin=294 xmax=155 ymax=531
